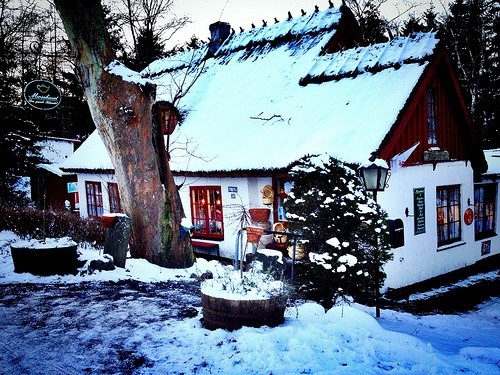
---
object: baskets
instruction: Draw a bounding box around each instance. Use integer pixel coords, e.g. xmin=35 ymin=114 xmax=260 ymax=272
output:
xmin=273 ymin=223 xmax=285 ymax=232
xmin=249 ymin=208 xmax=270 ymax=223
xmin=246 ymin=226 xmax=264 ymax=244
xmin=262 ymin=197 xmax=272 ymax=205
xmin=260 ymin=184 xmax=274 ymax=197
xmin=286 ymin=246 xmax=306 ymax=261
xmin=273 ymin=233 xmax=288 ymax=244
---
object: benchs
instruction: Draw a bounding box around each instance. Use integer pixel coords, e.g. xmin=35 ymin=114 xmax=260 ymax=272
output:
xmin=193 ymin=240 xmax=219 ymax=260
